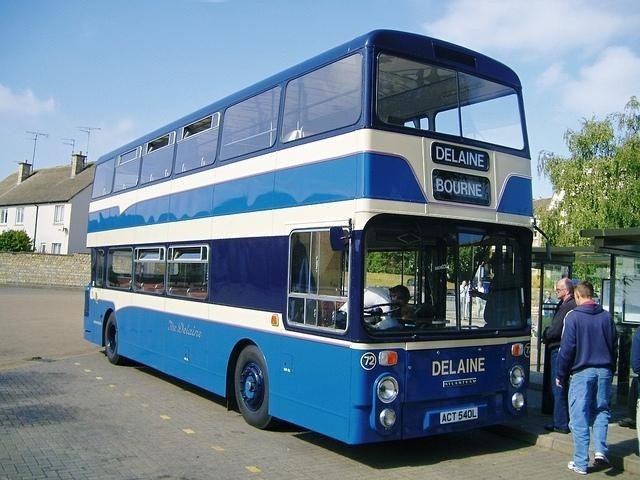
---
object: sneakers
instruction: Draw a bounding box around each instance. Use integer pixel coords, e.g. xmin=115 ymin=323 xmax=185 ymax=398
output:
xmin=594 ymin=452 xmax=609 ymax=464
xmin=568 ymin=460 xmax=586 ymax=475
xmin=545 ymin=424 xmax=567 ymax=434
xmin=619 ymin=418 xmax=632 ymax=426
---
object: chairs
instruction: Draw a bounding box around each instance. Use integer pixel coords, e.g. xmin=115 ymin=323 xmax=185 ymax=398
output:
xmin=117 ymin=278 xmax=207 ymax=299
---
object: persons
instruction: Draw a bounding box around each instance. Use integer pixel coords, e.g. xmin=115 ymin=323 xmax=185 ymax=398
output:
xmin=468 ymin=255 xmax=521 ymax=327
xmin=543 ymin=291 xmax=556 ymax=317
xmin=336 ymin=285 xmax=410 ymax=328
xmin=376 ymin=304 xmax=417 ymax=330
xmin=554 ymin=281 xmax=618 ymax=475
xmin=460 ymin=280 xmax=468 ymax=320
xmin=475 ymin=282 xmax=485 ymax=319
xmin=632 ymin=326 xmax=640 ymax=456
xmin=539 ymin=277 xmax=578 ymax=434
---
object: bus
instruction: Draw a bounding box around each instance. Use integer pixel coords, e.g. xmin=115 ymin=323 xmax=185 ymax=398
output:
xmin=83 ymin=29 xmax=537 ymax=445
